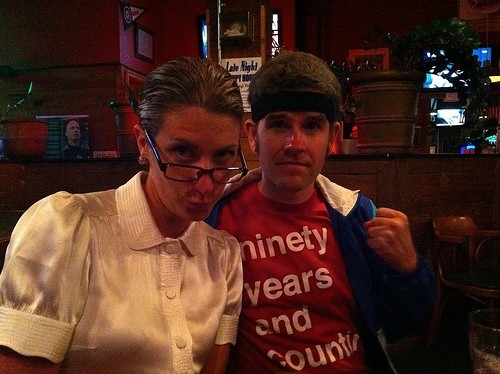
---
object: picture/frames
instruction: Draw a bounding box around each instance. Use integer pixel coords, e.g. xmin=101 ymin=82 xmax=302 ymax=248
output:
xmin=123 ymin=69 xmax=145 ymax=105
xmin=133 ymin=21 xmax=154 ymax=65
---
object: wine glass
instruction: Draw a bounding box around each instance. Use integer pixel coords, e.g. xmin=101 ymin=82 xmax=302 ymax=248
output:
xmin=0 ymin=122 xmax=48 ymax=213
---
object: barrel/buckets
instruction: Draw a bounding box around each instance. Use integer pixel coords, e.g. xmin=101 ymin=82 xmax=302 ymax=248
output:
xmin=350 ymin=79 xmax=419 ymax=152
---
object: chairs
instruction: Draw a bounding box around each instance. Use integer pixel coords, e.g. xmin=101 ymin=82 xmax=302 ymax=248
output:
xmin=425 ymin=215 xmax=500 ymax=350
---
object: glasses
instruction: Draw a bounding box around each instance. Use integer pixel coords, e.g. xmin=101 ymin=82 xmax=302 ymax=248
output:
xmin=145 ymin=129 xmax=248 ymax=184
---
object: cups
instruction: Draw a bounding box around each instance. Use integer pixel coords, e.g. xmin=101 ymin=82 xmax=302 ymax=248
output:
xmin=467 ymin=308 xmax=500 ymax=374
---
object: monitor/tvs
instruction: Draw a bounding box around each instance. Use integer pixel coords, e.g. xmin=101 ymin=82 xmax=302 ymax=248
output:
xmin=198 ymin=14 xmax=209 ymax=59
xmin=434 ymin=107 xmax=467 ymax=127
xmin=415 ymin=45 xmax=494 ymax=92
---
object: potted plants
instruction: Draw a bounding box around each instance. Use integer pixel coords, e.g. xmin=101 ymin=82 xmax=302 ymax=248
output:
xmin=0 ymin=80 xmax=55 ymax=159
xmin=109 ymin=73 xmax=144 ymax=157
xmin=342 ymin=15 xmax=499 ymax=155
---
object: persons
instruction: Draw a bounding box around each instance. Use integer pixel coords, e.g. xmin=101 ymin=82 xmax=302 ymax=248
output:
xmin=0 ymin=57 xmax=247 ymax=374
xmin=204 ymin=50 xmax=438 ymax=374
xmin=60 ymin=120 xmax=90 ymax=161
